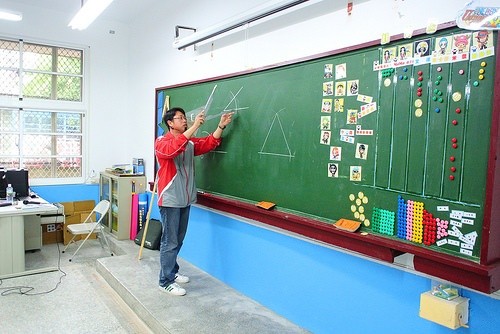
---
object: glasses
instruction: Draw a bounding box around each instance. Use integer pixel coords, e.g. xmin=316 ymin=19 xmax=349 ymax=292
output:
xmin=173 ymin=117 xmax=187 ymax=120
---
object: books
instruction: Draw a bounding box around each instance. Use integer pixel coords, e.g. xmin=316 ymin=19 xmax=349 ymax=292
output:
xmin=131 ymin=157 xmax=145 ymax=175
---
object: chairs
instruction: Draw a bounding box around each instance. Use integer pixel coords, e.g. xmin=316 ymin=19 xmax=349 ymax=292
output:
xmin=62 ymin=200 xmax=113 ymax=262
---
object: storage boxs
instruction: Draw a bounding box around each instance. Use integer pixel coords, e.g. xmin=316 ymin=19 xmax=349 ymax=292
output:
xmin=41 ymin=201 xmax=97 ymax=244
xmin=419 ymin=278 xmax=471 ymax=330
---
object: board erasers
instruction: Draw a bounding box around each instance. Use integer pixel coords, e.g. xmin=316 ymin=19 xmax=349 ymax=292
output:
xmin=333 ymin=216 xmax=362 ymax=232
xmin=257 ymin=201 xmax=276 ymax=210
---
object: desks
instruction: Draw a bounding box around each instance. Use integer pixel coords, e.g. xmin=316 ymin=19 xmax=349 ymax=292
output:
xmin=0 ymin=189 xmax=65 ymax=273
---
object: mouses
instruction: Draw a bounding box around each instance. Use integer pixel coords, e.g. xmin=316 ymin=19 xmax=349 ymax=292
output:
xmin=31 ymin=195 xmax=36 ymax=198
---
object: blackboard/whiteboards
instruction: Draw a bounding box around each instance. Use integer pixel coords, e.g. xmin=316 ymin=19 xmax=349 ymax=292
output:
xmin=148 ymin=21 xmax=500 ymax=292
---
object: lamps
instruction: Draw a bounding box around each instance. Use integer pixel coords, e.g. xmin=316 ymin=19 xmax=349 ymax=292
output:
xmin=67 ymin=0 xmax=112 ymax=31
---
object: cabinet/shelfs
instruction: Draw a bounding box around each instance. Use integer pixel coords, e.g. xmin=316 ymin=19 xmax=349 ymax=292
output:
xmin=99 ymin=172 xmax=146 ymax=241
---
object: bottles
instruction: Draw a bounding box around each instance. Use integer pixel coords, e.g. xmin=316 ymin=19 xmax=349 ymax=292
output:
xmin=6 ymin=184 xmax=13 ymax=202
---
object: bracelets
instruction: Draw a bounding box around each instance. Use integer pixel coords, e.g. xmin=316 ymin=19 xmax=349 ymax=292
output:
xmin=218 ymin=125 xmax=226 ymax=130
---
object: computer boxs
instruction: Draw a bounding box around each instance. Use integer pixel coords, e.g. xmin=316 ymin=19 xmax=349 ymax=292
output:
xmin=0 ymin=169 xmax=29 ymax=199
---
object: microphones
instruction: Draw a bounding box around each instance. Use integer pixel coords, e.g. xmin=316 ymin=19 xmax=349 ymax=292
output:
xmin=23 ymin=200 xmax=40 ymax=205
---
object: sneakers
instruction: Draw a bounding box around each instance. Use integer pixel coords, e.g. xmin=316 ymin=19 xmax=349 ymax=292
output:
xmin=173 ymin=273 xmax=189 ymax=283
xmin=159 ymin=282 xmax=186 ymax=296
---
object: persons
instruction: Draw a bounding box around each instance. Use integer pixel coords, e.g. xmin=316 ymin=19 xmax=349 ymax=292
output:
xmin=154 ymin=108 xmax=237 ymax=296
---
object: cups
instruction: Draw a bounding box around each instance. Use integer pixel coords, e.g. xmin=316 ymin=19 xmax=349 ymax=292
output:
xmin=18 ymin=201 xmax=23 ymax=206
